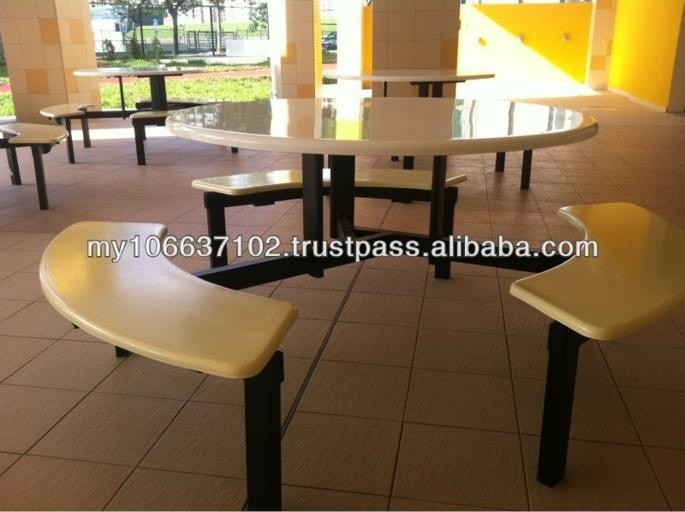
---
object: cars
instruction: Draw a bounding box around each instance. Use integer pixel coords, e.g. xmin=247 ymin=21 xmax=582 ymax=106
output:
xmin=113 ymin=16 xmax=142 ymax=32
xmin=322 ymin=30 xmax=338 ymax=52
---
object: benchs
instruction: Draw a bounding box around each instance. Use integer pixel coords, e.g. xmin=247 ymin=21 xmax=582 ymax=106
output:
xmin=0 ymin=96 xmax=239 ymax=210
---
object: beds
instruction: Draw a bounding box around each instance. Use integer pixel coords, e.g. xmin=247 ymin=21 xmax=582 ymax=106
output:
xmin=72 ymin=66 xmax=183 ymax=111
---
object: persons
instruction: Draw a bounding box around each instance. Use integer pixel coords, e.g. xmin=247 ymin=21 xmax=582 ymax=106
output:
xmin=153 ymin=18 xmax=159 ymax=36
xmin=120 ymin=17 xmax=128 ymax=38
